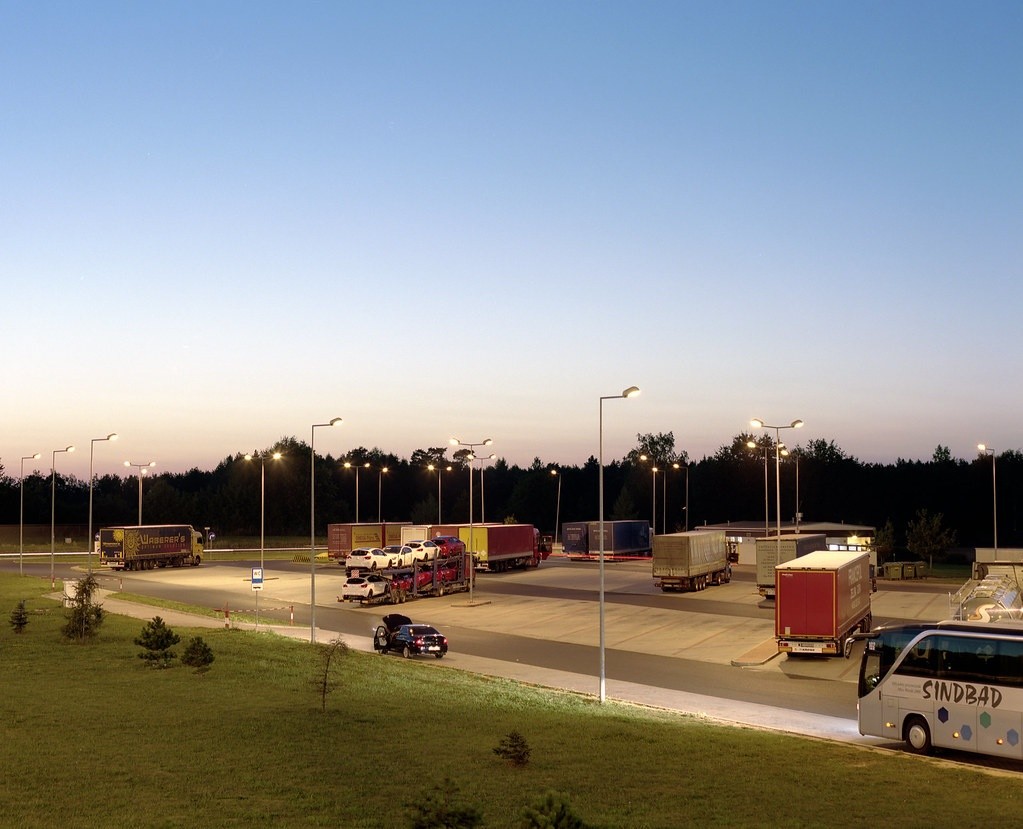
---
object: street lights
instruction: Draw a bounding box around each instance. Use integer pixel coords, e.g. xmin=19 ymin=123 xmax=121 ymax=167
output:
xmin=428 ymin=464 xmax=453 ymax=524
xmin=977 ymin=444 xmax=997 ymax=561
xmin=599 ymin=386 xmax=641 ymax=703
xmin=466 ymin=453 xmax=497 ymax=526
xmin=88 ymin=433 xmax=120 ymax=576
xmin=244 ymin=452 xmax=282 ymax=568
xmin=449 ymin=437 xmax=494 ymax=604
xmin=19 ymin=452 xmax=43 ymax=578
xmin=751 ymin=417 xmax=805 ymax=565
xmin=311 ymin=417 xmax=345 ymax=642
xmin=344 ymin=462 xmax=371 ymax=523
xmin=50 ymin=446 xmax=76 ymax=578
xmin=124 ymin=461 xmax=156 ymax=526
xmin=747 ymin=441 xmax=786 ymax=537
xmin=640 ymin=455 xmax=690 ymax=536
xmin=780 ymin=449 xmax=799 ymax=534
xmin=550 ymin=469 xmax=561 ymax=547
xmin=379 ymin=468 xmax=389 ymax=522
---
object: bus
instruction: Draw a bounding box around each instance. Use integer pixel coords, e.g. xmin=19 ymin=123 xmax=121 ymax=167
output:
xmin=843 ymin=619 xmax=1023 ymax=763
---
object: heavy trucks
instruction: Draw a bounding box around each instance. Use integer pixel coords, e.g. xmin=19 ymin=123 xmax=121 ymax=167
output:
xmin=327 ymin=521 xmax=553 ymax=607
xmin=99 ymin=524 xmax=205 ymax=573
xmin=561 ymin=519 xmax=652 ymax=562
xmin=775 ymin=548 xmax=878 ymax=661
xmin=652 ymin=529 xmax=735 ymax=592
xmin=755 ymin=533 xmax=830 ymax=600
xmin=948 ymin=547 xmax=1023 ymax=624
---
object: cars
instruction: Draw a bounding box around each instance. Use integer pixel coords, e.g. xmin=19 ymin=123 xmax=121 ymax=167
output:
xmin=373 ymin=613 xmax=448 ymax=658
